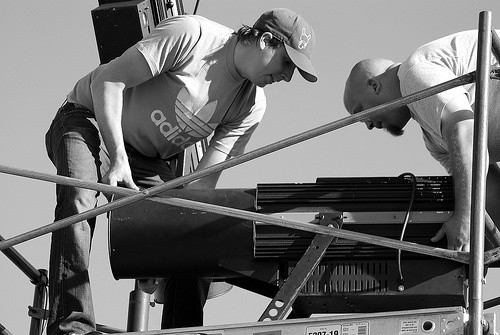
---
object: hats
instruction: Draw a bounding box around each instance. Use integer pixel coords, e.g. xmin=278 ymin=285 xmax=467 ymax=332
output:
xmin=252 ymin=8 xmax=317 ymax=83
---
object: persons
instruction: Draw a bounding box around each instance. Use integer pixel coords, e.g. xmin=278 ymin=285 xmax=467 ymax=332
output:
xmin=342 ymin=28 xmax=499 ymax=253
xmin=46 ymin=8 xmax=318 ymax=334
